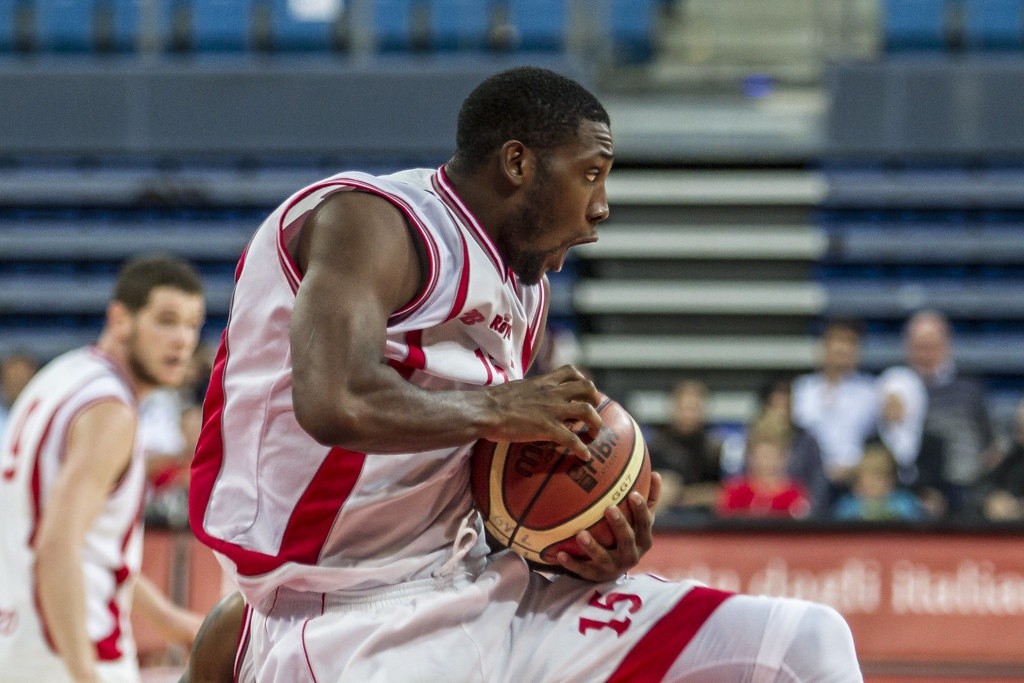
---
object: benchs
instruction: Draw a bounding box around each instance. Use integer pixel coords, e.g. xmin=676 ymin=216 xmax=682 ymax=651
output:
xmin=0 ymin=157 xmax=1023 ymax=480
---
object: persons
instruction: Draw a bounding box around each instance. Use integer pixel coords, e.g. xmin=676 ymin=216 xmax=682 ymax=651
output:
xmin=836 ymin=368 xmax=949 ymax=520
xmin=647 ymin=380 xmax=729 ymax=525
xmin=0 ymin=348 xmax=212 ymax=527
xmin=902 ymin=310 xmax=1015 ymax=524
xmin=790 ymin=326 xmax=884 ymax=482
xmin=978 ymin=407 xmax=1024 ymax=525
xmin=177 ymin=66 xmax=864 ymax=683
xmin=717 ymin=379 xmax=833 ymax=520
xmin=0 ymin=254 xmax=206 ymax=683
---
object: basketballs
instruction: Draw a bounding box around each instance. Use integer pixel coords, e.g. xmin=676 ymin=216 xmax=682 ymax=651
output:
xmin=469 ymin=386 xmax=651 ymax=572
